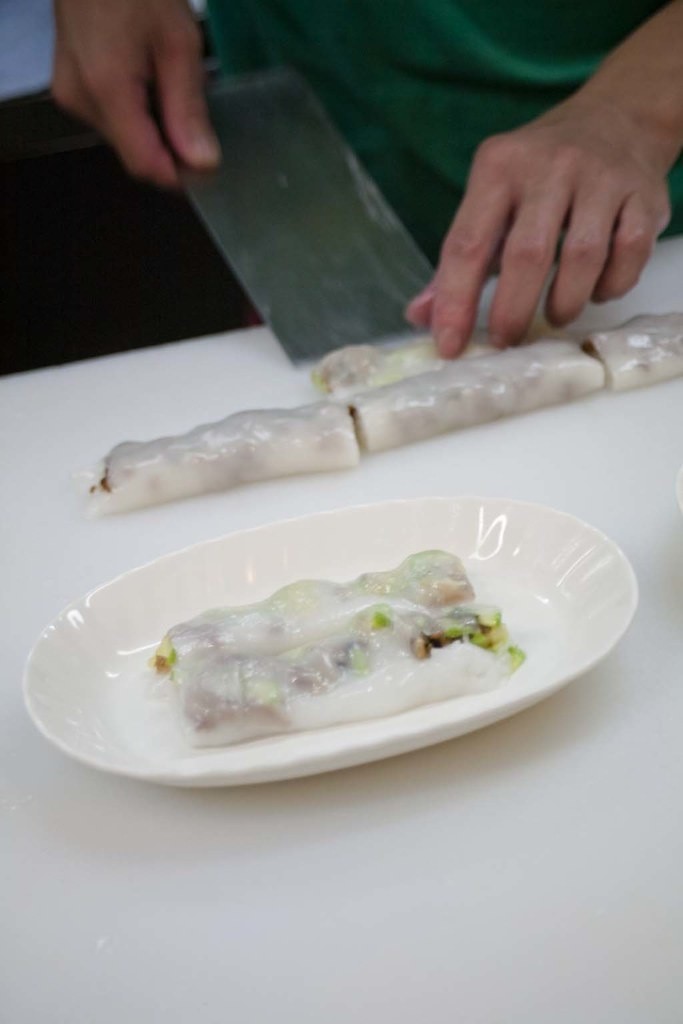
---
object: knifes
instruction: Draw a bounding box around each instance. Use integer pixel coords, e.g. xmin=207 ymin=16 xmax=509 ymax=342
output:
xmin=177 ymin=72 xmax=436 ymax=367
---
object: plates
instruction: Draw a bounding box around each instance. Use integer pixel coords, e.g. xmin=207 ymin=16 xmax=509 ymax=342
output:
xmin=23 ymin=497 xmax=638 ymax=787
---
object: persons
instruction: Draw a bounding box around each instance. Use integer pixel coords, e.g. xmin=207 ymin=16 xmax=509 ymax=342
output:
xmin=50 ymin=0 xmax=683 ymax=360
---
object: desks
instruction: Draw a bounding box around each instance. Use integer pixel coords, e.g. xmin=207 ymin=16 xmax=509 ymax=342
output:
xmin=0 ymin=237 xmax=682 ymax=1023
